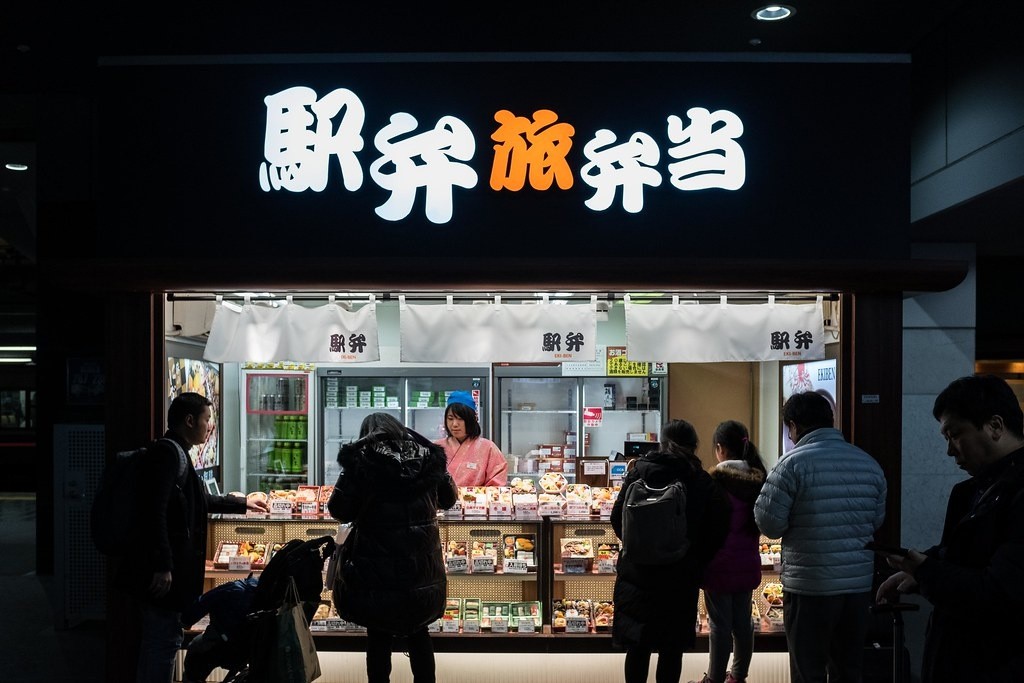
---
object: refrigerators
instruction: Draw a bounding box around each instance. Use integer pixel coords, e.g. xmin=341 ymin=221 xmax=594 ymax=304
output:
xmin=240 ymin=365 xmax=322 ymax=498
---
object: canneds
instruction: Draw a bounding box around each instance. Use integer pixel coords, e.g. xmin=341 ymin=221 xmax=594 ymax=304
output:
xmin=260 ymin=377 xmax=306 ymax=412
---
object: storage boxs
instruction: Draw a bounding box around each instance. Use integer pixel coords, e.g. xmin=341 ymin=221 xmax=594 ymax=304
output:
xmin=756 ymin=541 xmax=783 ymax=564
xmin=627 ymin=432 xmax=656 ymax=443
xmin=326 ymin=378 xmax=399 ymax=407
xmin=213 ymin=540 xmax=286 ymax=570
xmin=246 ymin=485 xmax=334 ymax=518
xmin=438 ymin=478 xmax=537 ymax=517
xmin=559 ymin=537 xmax=594 ymax=574
xmin=540 ymin=483 xmax=621 ymax=512
xmin=472 ymin=541 xmax=498 ymax=572
xmin=443 ymin=597 xmax=542 ymax=630
xmin=553 ymin=598 xmax=615 ymax=635
xmin=441 ymin=539 xmax=470 ymax=573
xmin=310 ymin=601 xmax=346 ymax=632
xmin=538 ymin=430 xmax=590 ymax=476
xmin=751 ymin=599 xmax=761 ymax=633
xmin=502 ymin=535 xmax=538 ymax=573
xmin=408 ymin=388 xmax=457 ymax=410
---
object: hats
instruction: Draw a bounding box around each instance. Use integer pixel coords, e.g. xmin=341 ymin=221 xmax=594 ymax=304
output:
xmin=447 ymin=390 xmax=477 ymax=410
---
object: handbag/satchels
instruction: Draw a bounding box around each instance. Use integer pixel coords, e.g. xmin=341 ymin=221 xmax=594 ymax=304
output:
xmin=326 ymin=543 xmax=343 ymax=590
xmin=275 ymin=577 xmax=321 ymax=683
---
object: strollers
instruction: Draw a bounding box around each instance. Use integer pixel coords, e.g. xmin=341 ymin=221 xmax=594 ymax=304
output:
xmin=200 ymin=534 xmax=338 ymax=683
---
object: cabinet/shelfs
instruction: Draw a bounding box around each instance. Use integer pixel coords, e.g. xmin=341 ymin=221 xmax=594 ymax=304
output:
xmin=318 ymin=367 xmax=494 ymax=490
xmin=494 ymin=360 xmax=578 ymax=486
xmin=433 ymin=521 xmax=543 ymax=653
xmin=586 ymin=377 xmax=664 ymax=489
xmin=208 ymin=520 xmax=367 ymax=651
xmin=545 ymin=511 xmax=623 ymax=654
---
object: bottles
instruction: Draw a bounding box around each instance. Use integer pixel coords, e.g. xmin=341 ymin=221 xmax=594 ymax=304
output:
xmin=259 ymin=476 xmax=307 ymax=494
xmin=273 ymin=415 xmax=307 ymax=439
xmin=257 ymin=440 xmax=306 ymax=474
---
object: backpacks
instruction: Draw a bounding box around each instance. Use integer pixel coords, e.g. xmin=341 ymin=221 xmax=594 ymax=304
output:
xmin=91 ymin=439 xmax=188 ymax=553
xmin=621 ymin=468 xmax=688 ymax=564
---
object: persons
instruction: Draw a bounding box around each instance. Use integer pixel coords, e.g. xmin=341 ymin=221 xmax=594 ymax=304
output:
xmin=426 ymin=389 xmax=508 ymax=487
xmin=756 ymin=392 xmax=887 ymax=683
xmin=689 ymin=421 xmax=766 ymax=683
xmin=121 ymin=393 xmax=268 ymax=683
xmin=327 ymin=412 xmax=459 ymax=683
xmin=875 ymin=374 xmax=1024 ymax=683
xmin=609 ymin=416 xmax=727 ymax=683
